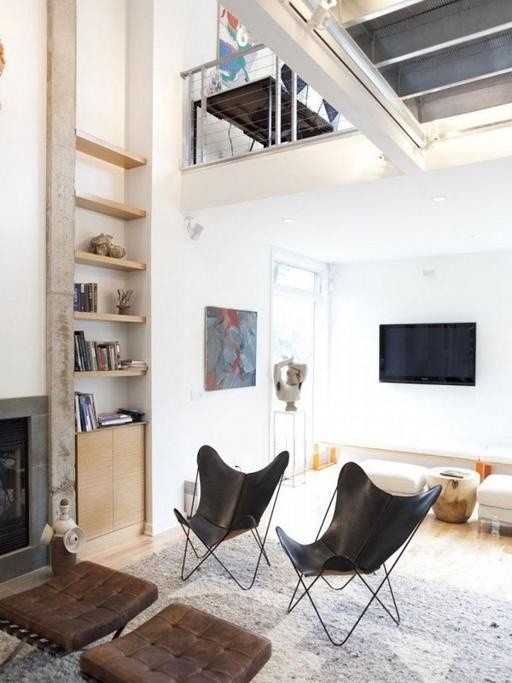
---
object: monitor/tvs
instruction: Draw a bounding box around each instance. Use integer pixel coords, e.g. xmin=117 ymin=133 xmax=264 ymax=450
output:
xmin=379 ymin=323 xmax=476 ymax=386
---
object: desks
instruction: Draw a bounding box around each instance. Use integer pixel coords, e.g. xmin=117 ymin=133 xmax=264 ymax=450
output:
xmin=425 ymin=466 xmax=480 ymax=525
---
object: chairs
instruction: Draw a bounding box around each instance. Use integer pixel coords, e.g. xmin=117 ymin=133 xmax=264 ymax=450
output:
xmin=275 ymin=461 xmax=442 ymax=646
xmin=173 ymin=445 xmax=289 ymax=591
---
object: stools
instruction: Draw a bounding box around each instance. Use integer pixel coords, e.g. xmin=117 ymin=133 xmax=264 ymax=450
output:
xmin=355 ymin=461 xmax=429 ymax=498
xmin=77 ymin=603 xmax=272 ymax=683
xmin=1 ymin=560 xmax=158 ymax=683
xmin=476 ymin=474 xmax=512 ymax=534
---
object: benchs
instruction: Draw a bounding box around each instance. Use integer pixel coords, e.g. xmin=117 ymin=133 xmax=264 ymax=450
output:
xmin=192 ymin=75 xmax=333 ymax=165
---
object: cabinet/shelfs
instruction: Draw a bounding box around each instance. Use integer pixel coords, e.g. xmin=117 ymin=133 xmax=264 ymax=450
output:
xmin=74 ymin=126 xmax=147 ymax=560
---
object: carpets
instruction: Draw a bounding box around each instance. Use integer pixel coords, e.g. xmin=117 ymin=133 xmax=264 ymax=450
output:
xmin=1 ymin=531 xmax=511 ymax=683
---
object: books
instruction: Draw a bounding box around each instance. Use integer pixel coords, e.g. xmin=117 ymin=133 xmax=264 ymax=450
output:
xmin=74 ymin=282 xmax=150 ymax=435
